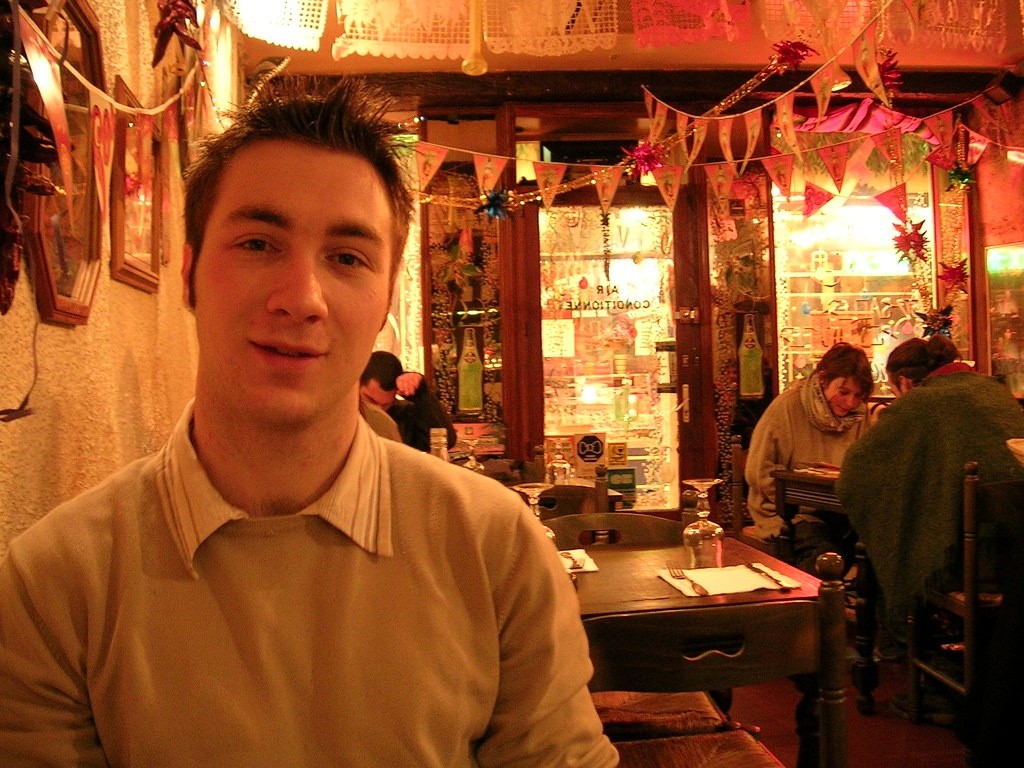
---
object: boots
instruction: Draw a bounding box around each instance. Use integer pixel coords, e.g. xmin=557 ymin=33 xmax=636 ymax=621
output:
xmin=889 ymin=671 xmax=963 ymax=726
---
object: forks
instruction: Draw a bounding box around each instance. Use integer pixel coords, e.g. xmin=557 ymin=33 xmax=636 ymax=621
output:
xmin=561 ymin=552 xmax=584 ymax=569
xmin=666 ymin=560 xmax=708 ymax=596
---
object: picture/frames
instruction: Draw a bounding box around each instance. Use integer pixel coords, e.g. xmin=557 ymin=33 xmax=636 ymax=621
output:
xmin=109 ymin=74 xmax=161 ymax=295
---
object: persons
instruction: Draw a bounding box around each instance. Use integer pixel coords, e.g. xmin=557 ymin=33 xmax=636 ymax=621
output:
xmin=745 ymin=336 xmax=1024 ymax=721
xmin=0 ymin=67 xmax=626 ymax=768
xmin=358 ymin=349 xmax=456 ymax=459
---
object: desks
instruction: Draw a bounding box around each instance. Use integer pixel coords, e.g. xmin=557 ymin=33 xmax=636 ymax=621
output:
xmin=554 ymin=478 xmax=626 ymax=544
xmin=769 ymin=469 xmax=882 ymax=717
xmin=559 ymin=534 xmax=821 ymax=768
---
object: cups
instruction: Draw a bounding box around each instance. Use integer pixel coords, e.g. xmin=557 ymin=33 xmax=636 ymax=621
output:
xmin=615 ymin=355 xmax=627 ymax=374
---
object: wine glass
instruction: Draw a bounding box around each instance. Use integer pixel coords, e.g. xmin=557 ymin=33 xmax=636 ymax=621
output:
xmin=512 ymin=483 xmax=555 ymax=544
xmin=456 ymin=438 xmax=484 ymax=475
xmin=682 ymin=478 xmax=723 ymax=569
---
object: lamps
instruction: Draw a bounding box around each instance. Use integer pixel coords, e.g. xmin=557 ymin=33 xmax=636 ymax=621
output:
xmin=817 ymin=23 xmax=852 ymax=92
xmin=460 ymin=0 xmax=489 ymax=76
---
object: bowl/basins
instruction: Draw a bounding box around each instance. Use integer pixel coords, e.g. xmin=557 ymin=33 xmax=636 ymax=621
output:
xmin=1006 ymin=439 xmax=1024 ymax=465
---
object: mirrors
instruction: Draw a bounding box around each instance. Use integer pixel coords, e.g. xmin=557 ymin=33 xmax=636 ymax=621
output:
xmin=20 ymin=0 xmax=107 ymax=328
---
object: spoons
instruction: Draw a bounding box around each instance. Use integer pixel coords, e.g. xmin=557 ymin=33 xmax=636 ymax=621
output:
xmin=745 ymin=562 xmax=801 ymax=591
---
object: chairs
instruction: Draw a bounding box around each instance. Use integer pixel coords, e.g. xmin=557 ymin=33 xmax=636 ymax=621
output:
xmin=906 ymin=460 xmax=1024 ymax=725
xmin=510 ymin=464 xmax=610 ymax=546
xmin=540 ymin=489 xmax=743 ymax=741
xmin=582 ymin=553 xmax=850 ymax=768
xmin=729 ymin=434 xmax=748 ymax=533
xmin=458 ymin=444 xmax=547 ymax=485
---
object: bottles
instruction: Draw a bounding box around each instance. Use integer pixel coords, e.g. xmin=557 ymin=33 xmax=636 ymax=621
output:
xmin=457 ymin=329 xmax=483 ymax=410
xmin=738 ymin=314 xmax=764 ymax=396
xmin=430 ymin=427 xmax=450 ymax=463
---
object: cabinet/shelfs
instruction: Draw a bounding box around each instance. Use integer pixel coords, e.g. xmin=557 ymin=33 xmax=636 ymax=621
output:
xmin=785 ymin=270 xmax=916 ymax=298
